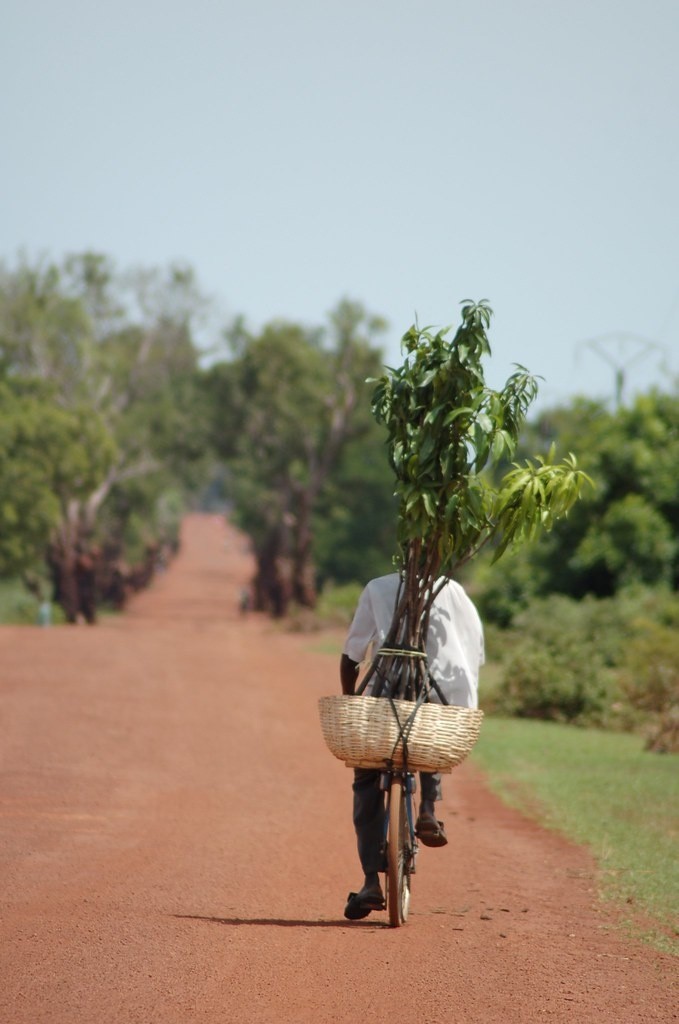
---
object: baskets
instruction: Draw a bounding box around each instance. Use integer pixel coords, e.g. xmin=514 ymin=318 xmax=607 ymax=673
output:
xmin=317 ymin=695 xmax=484 ymax=774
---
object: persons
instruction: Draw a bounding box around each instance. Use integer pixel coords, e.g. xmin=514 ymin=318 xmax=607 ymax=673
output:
xmin=339 ymin=570 xmax=487 ymax=921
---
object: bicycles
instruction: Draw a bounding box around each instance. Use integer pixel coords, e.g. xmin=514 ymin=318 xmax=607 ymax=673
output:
xmin=360 ymin=772 xmax=439 ymax=926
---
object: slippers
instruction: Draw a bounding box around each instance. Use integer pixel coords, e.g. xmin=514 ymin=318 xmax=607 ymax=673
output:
xmin=414 ymin=813 xmax=447 ymax=847
xmin=345 ymin=892 xmax=387 ymax=920
